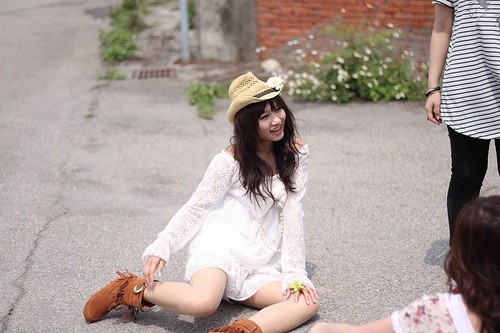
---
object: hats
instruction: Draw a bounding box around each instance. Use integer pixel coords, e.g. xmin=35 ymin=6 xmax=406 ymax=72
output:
xmin=227 ymin=72 xmax=284 ymax=125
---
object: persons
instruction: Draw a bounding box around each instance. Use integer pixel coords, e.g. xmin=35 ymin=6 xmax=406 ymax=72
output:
xmin=312 ymin=196 xmax=500 ymax=333
xmin=426 ymin=0 xmax=500 ymax=237
xmin=83 ymin=73 xmax=321 ymax=333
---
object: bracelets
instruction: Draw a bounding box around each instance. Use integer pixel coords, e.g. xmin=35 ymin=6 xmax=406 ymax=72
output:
xmin=426 ymin=86 xmax=440 ymax=96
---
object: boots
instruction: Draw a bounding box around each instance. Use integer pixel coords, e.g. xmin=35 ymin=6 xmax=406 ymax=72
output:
xmin=83 ymin=271 xmax=158 ymax=323
xmin=208 ymin=319 xmax=264 ymax=333
